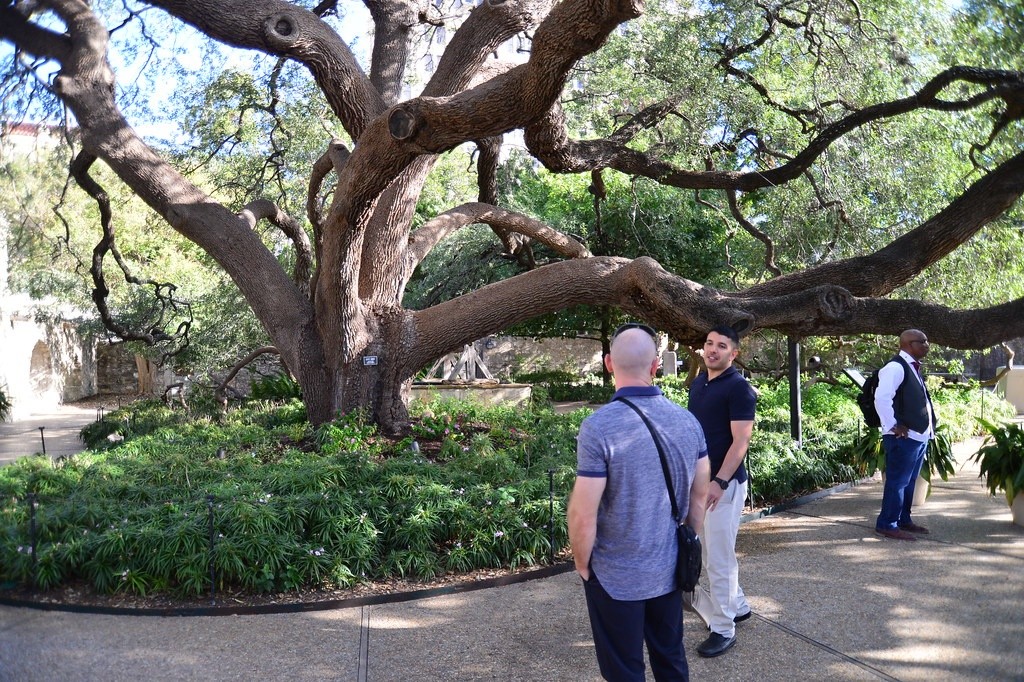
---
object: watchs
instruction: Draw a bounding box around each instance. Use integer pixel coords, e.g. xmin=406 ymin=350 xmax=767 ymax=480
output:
xmin=713 ymin=477 xmax=729 ymax=490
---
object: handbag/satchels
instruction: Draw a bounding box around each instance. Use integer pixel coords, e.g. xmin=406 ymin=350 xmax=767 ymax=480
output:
xmin=677 ymin=523 xmax=701 ymax=592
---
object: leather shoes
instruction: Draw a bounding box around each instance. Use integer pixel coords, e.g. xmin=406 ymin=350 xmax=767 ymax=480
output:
xmin=698 ymin=633 xmax=737 ymax=657
xmin=707 ymin=611 xmax=751 ymax=630
xmin=899 ymin=524 xmax=930 ymax=534
xmin=874 ymin=528 xmax=918 ymax=539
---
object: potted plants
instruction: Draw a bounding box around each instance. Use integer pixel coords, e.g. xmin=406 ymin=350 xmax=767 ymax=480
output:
xmin=853 ymin=418 xmax=959 ymax=507
xmin=968 ymin=415 xmax=1024 ymax=526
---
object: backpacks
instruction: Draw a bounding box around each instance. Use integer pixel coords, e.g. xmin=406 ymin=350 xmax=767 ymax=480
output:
xmin=857 ymin=359 xmax=909 ymax=427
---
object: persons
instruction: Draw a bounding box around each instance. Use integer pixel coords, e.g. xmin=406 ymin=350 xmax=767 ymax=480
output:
xmin=688 ymin=327 xmax=757 ymax=657
xmin=873 ymin=329 xmax=937 ymax=540
xmin=566 ymin=322 xmax=711 ymax=682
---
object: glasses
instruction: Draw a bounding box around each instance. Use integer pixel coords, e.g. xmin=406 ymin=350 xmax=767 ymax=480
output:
xmin=611 ymin=323 xmax=657 ymax=347
xmin=911 ymin=339 xmax=928 ymax=343
